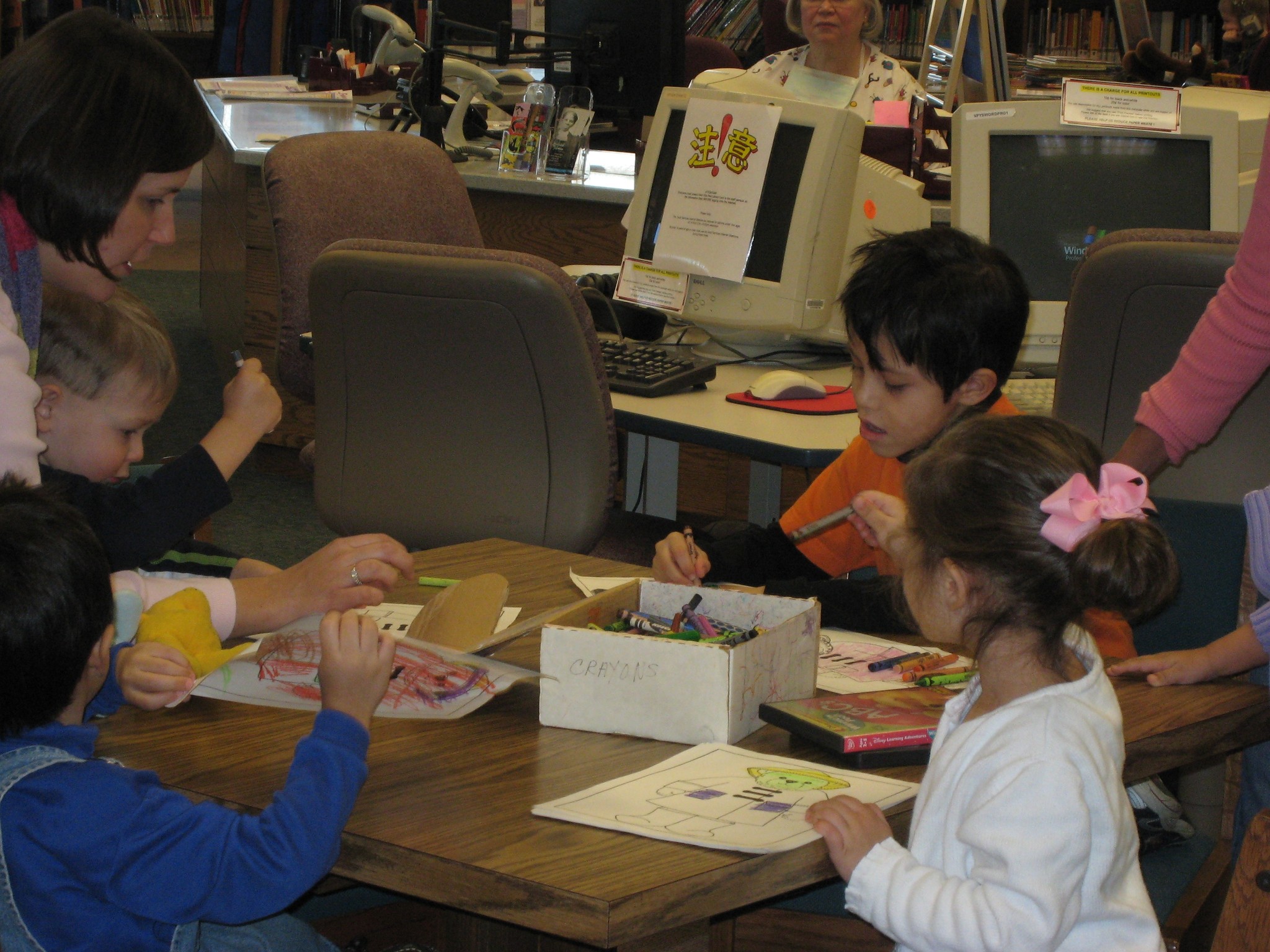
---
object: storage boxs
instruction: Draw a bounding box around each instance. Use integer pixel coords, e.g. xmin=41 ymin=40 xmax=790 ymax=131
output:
xmin=463 ymin=574 xmax=821 ymax=746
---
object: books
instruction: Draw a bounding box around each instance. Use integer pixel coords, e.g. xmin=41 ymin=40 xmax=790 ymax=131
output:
xmin=1 ymin=0 xmax=1269 ymax=125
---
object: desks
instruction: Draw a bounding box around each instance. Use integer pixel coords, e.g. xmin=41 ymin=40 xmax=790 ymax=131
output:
xmin=90 ymin=537 xmax=1270 ymax=952
xmin=561 ymin=261 xmax=1069 ymax=474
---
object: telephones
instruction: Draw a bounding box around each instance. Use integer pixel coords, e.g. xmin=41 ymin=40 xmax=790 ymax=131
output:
xmin=396 ymin=80 xmax=488 ymax=140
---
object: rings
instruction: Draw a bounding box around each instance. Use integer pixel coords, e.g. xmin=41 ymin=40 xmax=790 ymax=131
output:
xmin=350 ymin=564 xmax=363 ymax=586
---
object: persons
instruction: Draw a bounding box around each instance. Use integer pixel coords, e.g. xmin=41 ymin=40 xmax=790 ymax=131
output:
xmin=1099 ymin=488 xmax=1270 ymax=839
xmin=0 ymin=465 xmax=402 ymax=951
xmin=738 ymin=0 xmax=930 ymax=161
xmin=1111 ymin=134 xmax=1269 ymax=482
xmin=791 ymin=401 xmax=1173 ymax=951
xmin=658 ymin=222 xmax=1139 ymax=676
xmin=18 ymin=236 xmax=288 ymax=581
xmin=1 ymin=5 xmax=418 ymax=659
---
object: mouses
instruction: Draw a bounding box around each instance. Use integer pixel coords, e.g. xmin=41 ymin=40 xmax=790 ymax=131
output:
xmin=744 ymin=370 xmax=828 ymax=400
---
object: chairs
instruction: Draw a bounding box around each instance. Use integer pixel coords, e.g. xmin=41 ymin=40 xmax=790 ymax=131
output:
xmin=707 ymin=496 xmax=1258 ymax=952
xmin=295 ymin=235 xmax=699 ymax=568
xmin=1051 ymin=228 xmax=1269 ymax=506
xmin=684 ymin=34 xmax=745 ymax=87
xmin=260 ymin=131 xmax=484 ymax=470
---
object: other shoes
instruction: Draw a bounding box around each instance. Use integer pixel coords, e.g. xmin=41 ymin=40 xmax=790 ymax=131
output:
xmin=1256 ymin=869 xmax=1270 ymax=892
xmin=1126 ymin=776 xmax=1196 ymax=843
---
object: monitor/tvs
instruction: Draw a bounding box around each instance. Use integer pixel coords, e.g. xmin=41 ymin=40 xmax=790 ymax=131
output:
xmin=1177 ymin=86 xmax=1270 ymax=234
xmin=693 ymin=67 xmax=925 ymax=198
xmin=623 ymin=85 xmax=931 ymax=362
xmin=945 ymin=101 xmax=1242 ymax=378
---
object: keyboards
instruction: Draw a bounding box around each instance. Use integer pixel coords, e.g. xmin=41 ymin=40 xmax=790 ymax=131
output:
xmin=596 ymin=338 xmax=716 ymax=396
xmin=999 ymin=377 xmax=1055 ymax=421
